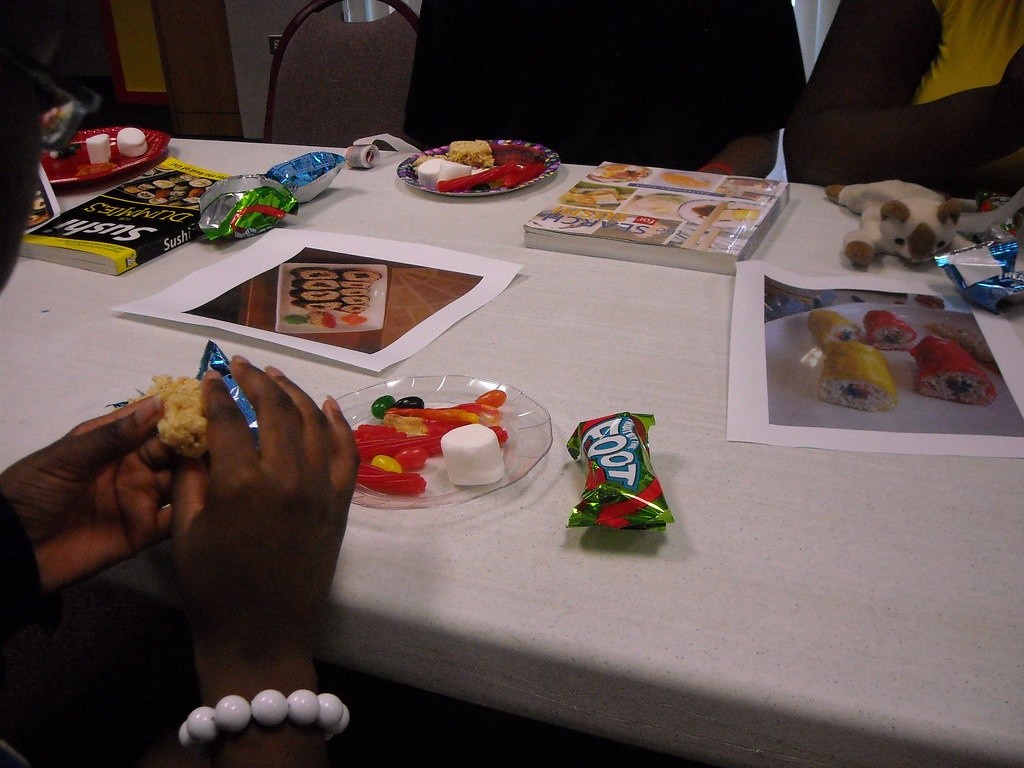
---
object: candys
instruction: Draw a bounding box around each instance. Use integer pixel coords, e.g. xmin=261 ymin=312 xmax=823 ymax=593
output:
xmin=566 ymin=412 xmax=675 ymax=530
xmin=348 ymin=390 xmax=508 ymax=494
xmin=140 ymin=372 xmax=211 ymax=458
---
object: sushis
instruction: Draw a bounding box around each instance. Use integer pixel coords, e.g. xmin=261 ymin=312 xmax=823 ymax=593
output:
xmin=289 ymin=267 xmax=382 ymax=315
xmin=808 ymin=307 xmax=997 ymax=412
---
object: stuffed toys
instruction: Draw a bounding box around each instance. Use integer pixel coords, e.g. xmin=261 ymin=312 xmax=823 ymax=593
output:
xmin=825 ymin=181 xmax=964 ymax=269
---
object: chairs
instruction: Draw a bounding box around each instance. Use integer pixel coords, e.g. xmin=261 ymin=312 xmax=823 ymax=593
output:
xmin=264 ymin=0 xmax=418 ymax=152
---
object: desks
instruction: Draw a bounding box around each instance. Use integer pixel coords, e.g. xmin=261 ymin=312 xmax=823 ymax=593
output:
xmin=0 ymin=135 xmax=1024 ymax=768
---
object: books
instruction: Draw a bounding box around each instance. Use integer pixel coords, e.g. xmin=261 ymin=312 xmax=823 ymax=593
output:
xmin=17 ymin=156 xmax=235 ymax=277
xmin=523 ymin=160 xmax=791 ymax=278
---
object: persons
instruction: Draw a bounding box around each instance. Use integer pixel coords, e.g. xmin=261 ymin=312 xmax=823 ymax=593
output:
xmin=0 ymin=60 xmax=359 ymax=768
xmin=403 ymin=0 xmax=805 ymax=180
xmin=782 ymin=0 xmax=1024 ymax=196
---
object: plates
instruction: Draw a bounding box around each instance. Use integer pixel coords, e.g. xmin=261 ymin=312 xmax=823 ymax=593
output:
xmin=573 ymin=187 xmax=619 ymax=200
xmin=335 ymin=375 xmax=553 ymax=510
xmin=276 ymin=263 xmax=388 ymax=334
xmin=679 ymin=200 xmax=767 ymax=227
xmin=764 ymin=305 xmax=1024 ymax=438
xmin=587 ymin=165 xmax=653 ymax=183
xmin=397 ymin=140 xmax=559 ymax=197
xmin=39 ymin=127 xmax=171 ymax=186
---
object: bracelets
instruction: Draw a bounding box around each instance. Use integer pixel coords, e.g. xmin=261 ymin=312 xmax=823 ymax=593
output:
xmin=700 ymin=161 xmax=736 ymax=176
xmin=180 ymin=689 xmax=352 ymax=748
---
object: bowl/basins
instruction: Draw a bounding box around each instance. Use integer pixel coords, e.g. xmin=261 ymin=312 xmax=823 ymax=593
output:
xmin=635 ymin=193 xmax=684 ymax=213
